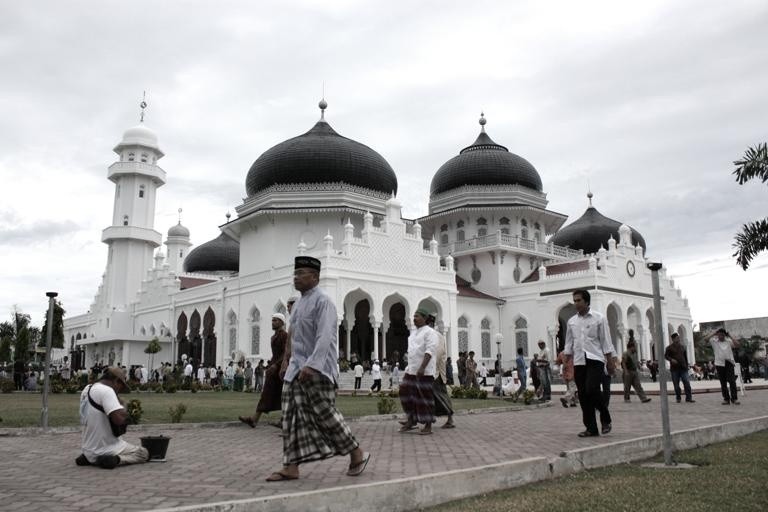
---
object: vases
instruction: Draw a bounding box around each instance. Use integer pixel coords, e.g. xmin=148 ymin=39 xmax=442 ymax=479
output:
xmin=139 ymin=435 xmax=173 ymax=462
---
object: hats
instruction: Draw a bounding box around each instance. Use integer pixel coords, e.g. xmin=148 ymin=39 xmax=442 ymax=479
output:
xmin=103 ymin=366 xmax=131 ymax=394
xmin=415 ymin=308 xmax=436 ymax=322
xmin=293 ymin=256 xmax=321 ymax=270
xmin=537 ymin=338 xmax=546 ymax=344
xmin=374 ymin=360 xmax=380 ymax=363
xmin=287 ymin=294 xmax=300 ymax=302
xmin=395 ymin=362 xmax=400 ymax=366
xmin=671 ymin=332 xmax=678 ymax=339
xmin=271 ymin=312 xmax=286 ymax=324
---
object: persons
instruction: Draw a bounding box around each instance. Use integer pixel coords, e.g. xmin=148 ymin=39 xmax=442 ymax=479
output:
xmin=561 ymin=290 xmax=615 ymax=438
xmin=614 ymin=357 xmax=767 ymax=383
xmin=287 ymin=294 xmax=298 ymax=313
xmin=266 ymin=257 xmax=370 ymax=482
xmin=445 ymin=340 xmax=578 ymax=408
xmin=339 ymin=346 xmax=409 ymax=394
xmin=397 ymin=310 xmax=439 ymax=435
xmin=600 ymin=343 xmax=617 ymax=406
xmin=237 ymin=313 xmax=289 ymax=428
xmin=704 ymin=328 xmax=741 ymax=405
xmin=75 ymin=367 xmax=150 ymax=469
xmin=664 ymin=334 xmax=696 ymax=403
xmin=11 ymin=355 xmax=266 ymax=395
xmin=398 ymin=315 xmax=457 ymax=429
xmin=621 ymin=342 xmax=651 ymax=403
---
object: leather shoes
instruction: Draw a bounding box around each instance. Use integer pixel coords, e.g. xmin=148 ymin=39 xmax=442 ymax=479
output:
xmin=570 ymin=404 xmax=577 ymax=407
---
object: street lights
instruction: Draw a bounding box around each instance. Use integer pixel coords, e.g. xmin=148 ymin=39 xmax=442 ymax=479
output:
xmin=494 ymin=333 xmax=504 ymax=401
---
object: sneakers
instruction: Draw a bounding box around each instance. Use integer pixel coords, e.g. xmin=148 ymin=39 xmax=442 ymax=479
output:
xmin=238 ymin=415 xmax=257 ymax=428
xmin=75 ymin=453 xmax=94 ymax=466
xmin=559 ymin=398 xmax=569 ymax=408
xmin=368 ymin=387 xmax=373 ymax=394
xmin=676 ymin=399 xmax=696 ymax=402
xmin=731 ymin=398 xmax=740 ymax=405
xmin=641 ymin=397 xmax=652 ymax=403
xmin=96 ymin=456 xmax=119 ymax=469
xmin=268 ymin=418 xmax=283 ymax=429
xmin=720 ymin=398 xmax=730 ymax=405
xmin=538 ymin=396 xmax=551 ymax=401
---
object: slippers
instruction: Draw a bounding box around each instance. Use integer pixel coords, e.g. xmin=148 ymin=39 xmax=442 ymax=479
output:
xmin=346 ymin=451 xmax=370 ymax=476
xmin=265 ymin=471 xmax=300 ymax=482
xmin=399 ymin=419 xmax=455 ymax=434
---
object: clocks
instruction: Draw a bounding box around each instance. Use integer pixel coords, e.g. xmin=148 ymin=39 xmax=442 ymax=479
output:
xmin=627 ymin=260 xmax=635 ymax=277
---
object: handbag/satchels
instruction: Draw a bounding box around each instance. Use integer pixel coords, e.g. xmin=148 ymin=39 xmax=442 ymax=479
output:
xmin=108 ymin=413 xmax=128 ymax=437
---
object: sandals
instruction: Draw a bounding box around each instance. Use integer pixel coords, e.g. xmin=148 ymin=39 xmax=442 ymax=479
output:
xmin=577 ymin=428 xmax=599 ymax=437
xmin=601 ymin=414 xmax=611 ymax=433
